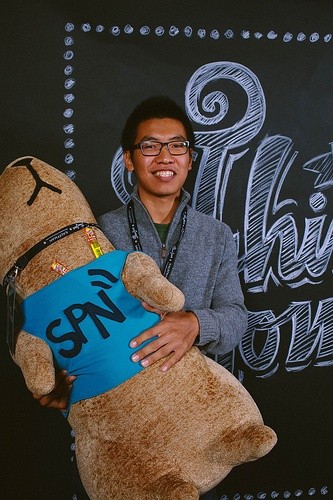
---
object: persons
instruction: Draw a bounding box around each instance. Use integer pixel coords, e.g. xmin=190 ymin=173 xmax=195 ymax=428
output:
xmin=32 ymin=95 xmax=248 ymax=499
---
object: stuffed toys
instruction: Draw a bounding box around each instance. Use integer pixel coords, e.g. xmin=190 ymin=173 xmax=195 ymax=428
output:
xmin=0 ymin=156 xmax=277 ymax=500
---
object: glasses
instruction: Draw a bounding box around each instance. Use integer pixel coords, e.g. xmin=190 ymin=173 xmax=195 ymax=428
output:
xmin=129 ymin=140 xmax=190 ymax=156
